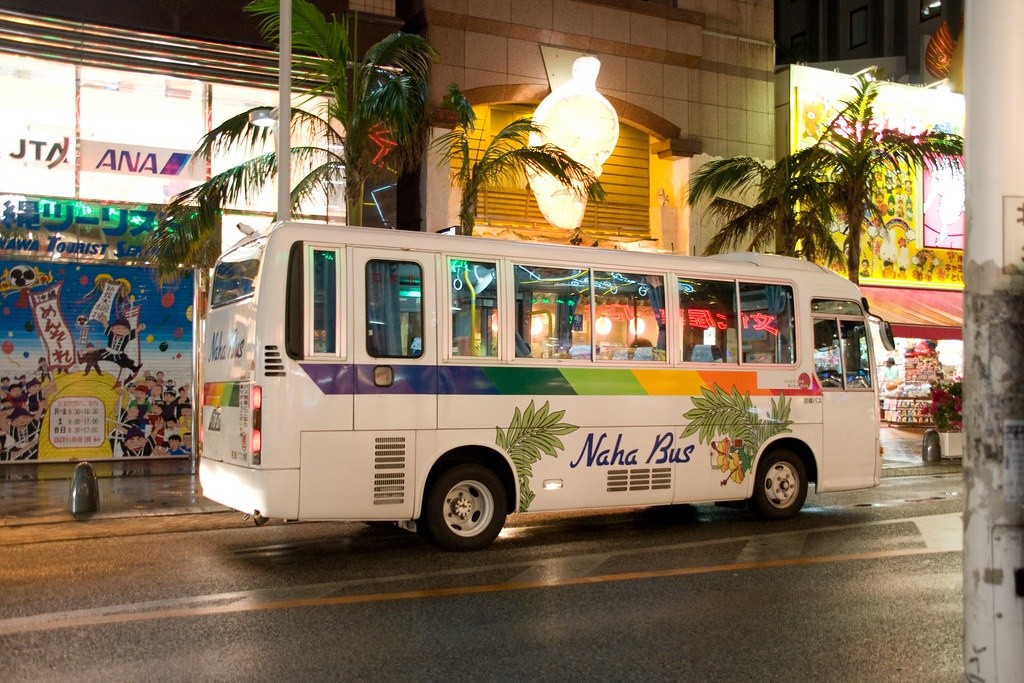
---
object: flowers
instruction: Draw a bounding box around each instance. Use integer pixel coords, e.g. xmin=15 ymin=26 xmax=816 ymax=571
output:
xmin=921 ymin=377 xmax=962 ymax=431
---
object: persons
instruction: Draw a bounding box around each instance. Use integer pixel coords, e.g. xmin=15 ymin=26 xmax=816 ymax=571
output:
xmin=885 ymin=357 xmax=903 ymax=381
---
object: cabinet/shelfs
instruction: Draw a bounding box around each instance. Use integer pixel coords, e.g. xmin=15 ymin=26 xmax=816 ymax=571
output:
xmin=882 ymin=395 xmax=934 ymax=427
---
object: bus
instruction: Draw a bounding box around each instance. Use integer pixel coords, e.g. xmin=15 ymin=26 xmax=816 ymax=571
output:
xmin=186 ymin=218 xmax=896 ymax=555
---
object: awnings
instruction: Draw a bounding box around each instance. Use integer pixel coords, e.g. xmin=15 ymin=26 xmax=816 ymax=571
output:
xmin=859 ymin=285 xmax=963 ymax=341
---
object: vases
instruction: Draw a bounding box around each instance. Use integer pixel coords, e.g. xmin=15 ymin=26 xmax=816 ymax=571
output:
xmin=937 ymin=432 xmax=962 ymax=459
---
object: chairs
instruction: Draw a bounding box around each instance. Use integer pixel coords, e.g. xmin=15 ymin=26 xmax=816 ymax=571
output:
xmin=568 ymin=344 xmax=722 ymax=362
xmin=745 ymin=350 xmax=774 ymax=363
